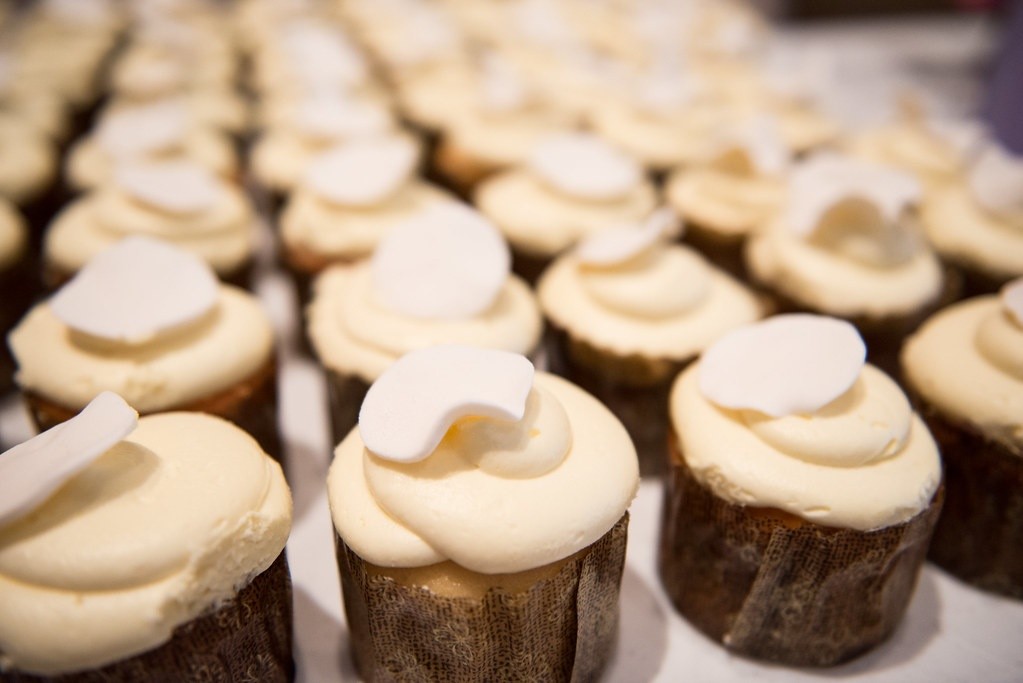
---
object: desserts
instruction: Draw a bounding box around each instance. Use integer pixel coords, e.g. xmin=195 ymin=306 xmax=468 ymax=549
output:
xmin=0 ymin=0 xmax=1023 ymax=683
